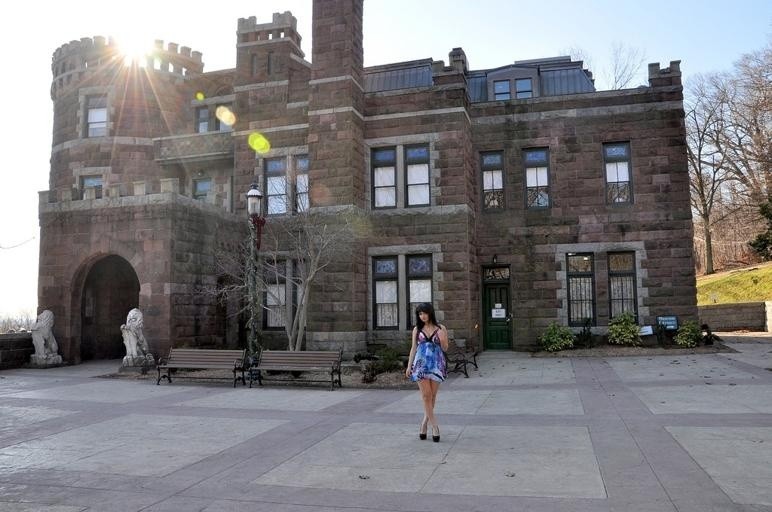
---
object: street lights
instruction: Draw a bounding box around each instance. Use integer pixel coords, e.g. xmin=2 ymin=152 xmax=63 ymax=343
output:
xmin=233 ymin=183 xmax=263 ymax=387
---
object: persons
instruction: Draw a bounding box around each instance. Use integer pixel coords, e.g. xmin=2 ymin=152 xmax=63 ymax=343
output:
xmin=406 ymin=302 xmax=449 ymax=442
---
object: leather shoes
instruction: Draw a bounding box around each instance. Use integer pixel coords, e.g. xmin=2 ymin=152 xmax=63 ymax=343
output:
xmin=433 ymin=435 xmax=440 ymax=442
xmin=420 ymin=434 xmax=426 ymax=440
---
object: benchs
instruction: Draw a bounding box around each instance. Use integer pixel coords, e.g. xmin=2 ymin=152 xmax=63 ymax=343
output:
xmin=155 ymin=345 xmax=343 ymax=394
xmin=440 ymin=338 xmax=482 ymax=379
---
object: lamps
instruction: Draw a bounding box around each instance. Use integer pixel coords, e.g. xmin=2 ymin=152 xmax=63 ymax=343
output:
xmin=492 ymin=253 xmax=498 ymax=265
